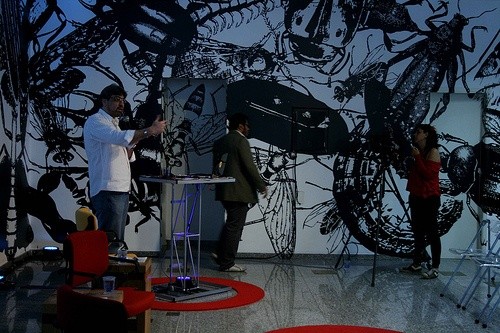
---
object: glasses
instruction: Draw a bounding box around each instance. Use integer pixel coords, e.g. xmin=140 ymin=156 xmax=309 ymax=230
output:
xmin=241 ymin=123 xmax=249 ymax=128
xmin=109 ymin=96 xmax=126 ymax=102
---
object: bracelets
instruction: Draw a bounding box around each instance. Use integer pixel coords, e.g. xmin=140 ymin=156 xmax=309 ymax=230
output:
xmin=144 ymin=128 xmax=148 ymax=136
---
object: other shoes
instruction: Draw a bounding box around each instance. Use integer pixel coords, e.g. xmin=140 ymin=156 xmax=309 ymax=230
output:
xmin=400 ymin=264 xmax=422 ymax=275
xmin=420 ymin=270 xmax=439 ymax=279
xmin=209 ymin=253 xmax=219 ymax=263
xmin=223 ymin=265 xmax=247 ymax=272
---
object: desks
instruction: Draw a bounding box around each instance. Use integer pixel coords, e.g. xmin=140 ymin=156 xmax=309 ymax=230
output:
xmin=140 ymin=175 xmax=236 ymax=292
xmin=43 ymin=289 xmax=123 ymax=313
xmin=105 ymin=253 xmax=152 ymax=294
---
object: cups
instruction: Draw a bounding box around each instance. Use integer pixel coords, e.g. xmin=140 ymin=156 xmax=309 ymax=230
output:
xmin=102 ymin=276 xmax=116 ymax=295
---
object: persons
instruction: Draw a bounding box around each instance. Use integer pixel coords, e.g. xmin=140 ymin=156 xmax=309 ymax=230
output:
xmin=84 ymin=83 xmax=170 ymax=241
xmin=401 ymin=124 xmax=441 ymax=279
xmin=212 ymin=114 xmax=267 ymax=271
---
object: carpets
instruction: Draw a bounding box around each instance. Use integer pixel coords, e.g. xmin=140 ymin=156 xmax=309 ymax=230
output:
xmin=150 ymin=276 xmax=265 ymax=311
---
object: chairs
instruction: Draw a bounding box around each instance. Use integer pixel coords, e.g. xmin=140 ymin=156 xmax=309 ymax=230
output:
xmin=56 ymin=285 xmax=129 ymax=333
xmin=63 ymin=231 xmax=154 ymax=333
xmin=75 ymin=206 xmax=139 ymax=286
xmin=439 ymin=218 xmax=500 ymax=329
